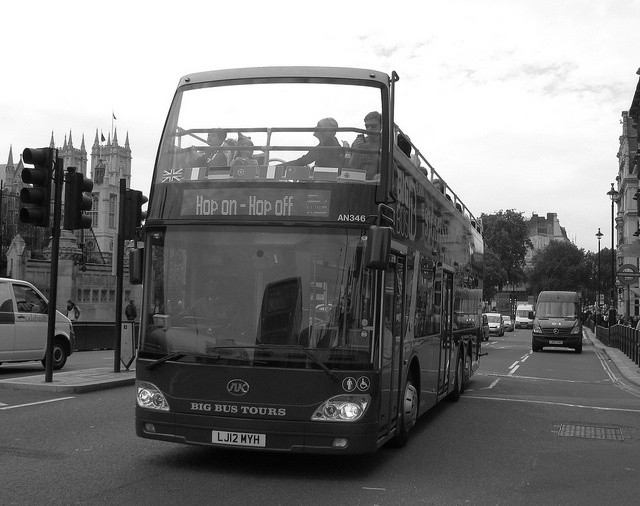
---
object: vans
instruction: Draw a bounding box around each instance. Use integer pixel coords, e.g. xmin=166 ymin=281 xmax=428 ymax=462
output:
xmin=515 ymin=304 xmax=535 ymax=328
xmin=0 ymin=278 xmax=75 ymax=369
xmin=533 ymin=290 xmax=582 ymax=353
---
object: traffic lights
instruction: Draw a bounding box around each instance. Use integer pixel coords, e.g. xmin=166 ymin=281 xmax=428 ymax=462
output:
xmin=17 ymin=146 xmax=53 ymax=227
xmin=124 ymin=188 xmax=148 ymax=240
xmin=64 ymin=171 xmax=94 ymax=230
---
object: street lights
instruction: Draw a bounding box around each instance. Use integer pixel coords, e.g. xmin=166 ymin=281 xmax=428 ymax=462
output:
xmin=607 ymin=182 xmax=620 ymax=324
xmin=596 ymin=228 xmax=604 ymax=325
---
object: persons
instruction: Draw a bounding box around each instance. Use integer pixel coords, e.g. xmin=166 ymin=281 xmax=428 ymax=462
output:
xmin=230 ymin=129 xmax=259 ymax=178
xmin=167 ymin=276 xmax=230 ymax=327
xmin=125 ymin=300 xmax=137 ymax=320
xmin=66 ymin=299 xmax=80 ymax=321
xmin=347 ymin=111 xmax=384 ymax=180
xmin=182 ymin=129 xmax=227 ymax=180
xmin=274 ymin=117 xmax=344 ymax=176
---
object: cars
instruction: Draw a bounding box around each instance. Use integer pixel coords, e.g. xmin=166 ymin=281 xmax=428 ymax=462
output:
xmin=485 ymin=312 xmax=504 ymax=336
xmin=502 ymin=315 xmax=514 ymax=331
xmin=482 ymin=314 xmax=490 ymax=341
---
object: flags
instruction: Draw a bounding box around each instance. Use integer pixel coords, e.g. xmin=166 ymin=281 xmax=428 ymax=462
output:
xmin=101 ymin=133 xmax=105 ymax=142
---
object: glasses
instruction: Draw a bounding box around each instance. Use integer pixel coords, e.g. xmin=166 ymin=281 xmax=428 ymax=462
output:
xmin=365 ymin=123 xmax=378 ymax=128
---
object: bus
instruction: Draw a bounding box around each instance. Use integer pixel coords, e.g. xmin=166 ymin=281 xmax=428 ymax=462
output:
xmin=249 ymin=274 xmax=305 ymax=365
xmin=129 ymin=65 xmax=486 ymax=455
